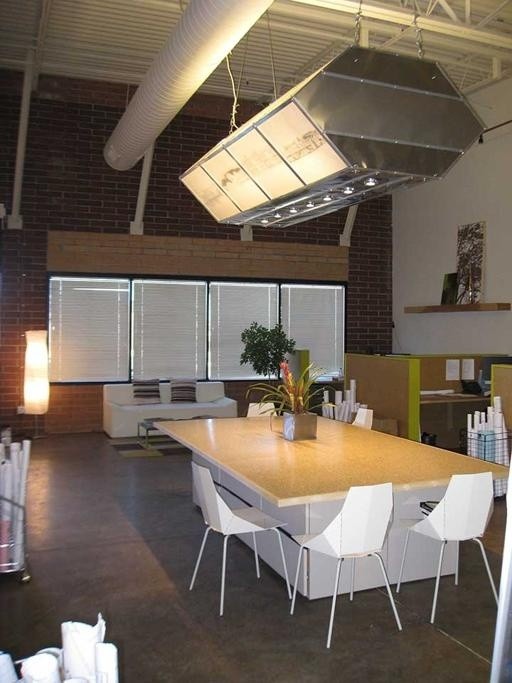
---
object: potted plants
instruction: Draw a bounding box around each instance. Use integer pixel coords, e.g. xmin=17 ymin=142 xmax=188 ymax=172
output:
xmin=280 ymin=403 xmax=318 ymax=441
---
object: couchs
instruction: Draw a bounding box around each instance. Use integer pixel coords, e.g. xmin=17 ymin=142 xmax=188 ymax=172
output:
xmin=102 ymin=381 xmax=238 ymax=439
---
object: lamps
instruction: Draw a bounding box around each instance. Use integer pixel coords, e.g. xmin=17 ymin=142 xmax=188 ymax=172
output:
xmin=23 ymin=330 xmax=50 ymax=440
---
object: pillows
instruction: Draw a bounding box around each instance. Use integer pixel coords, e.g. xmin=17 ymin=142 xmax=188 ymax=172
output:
xmin=170 ymin=377 xmax=198 ymax=403
xmin=131 ymin=378 xmax=162 ymax=405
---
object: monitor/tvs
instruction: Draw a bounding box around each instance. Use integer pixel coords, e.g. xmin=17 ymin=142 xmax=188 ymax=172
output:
xmin=484 ymin=356 xmax=512 ymax=384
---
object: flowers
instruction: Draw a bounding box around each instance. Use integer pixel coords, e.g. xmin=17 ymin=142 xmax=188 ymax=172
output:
xmin=245 ymin=362 xmax=339 ymax=431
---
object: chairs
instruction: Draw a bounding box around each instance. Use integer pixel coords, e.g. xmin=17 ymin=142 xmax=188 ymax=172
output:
xmin=190 ymin=462 xmax=293 ymax=616
xmin=397 ymin=472 xmax=500 ymax=624
xmin=289 ymin=482 xmax=403 ymax=647
xmin=246 ymin=403 xmax=373 ymax=429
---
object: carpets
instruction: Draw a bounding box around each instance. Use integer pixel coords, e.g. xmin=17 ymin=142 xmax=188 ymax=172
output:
xmin=107 ymin=434 xmax=192 ymax=460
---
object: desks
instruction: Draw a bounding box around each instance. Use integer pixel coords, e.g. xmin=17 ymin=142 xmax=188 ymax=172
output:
xmin=420 ymin=391 xmax=492 ymax=405
xmin=152 ymin=414 xmax=510 ymax=601
xmin=153 ymin=414 xmax=500 ymax=600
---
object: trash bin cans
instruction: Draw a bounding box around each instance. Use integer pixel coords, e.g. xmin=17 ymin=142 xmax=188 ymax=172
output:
xmin=421 ymin=432 xmax=437 ymax=446
